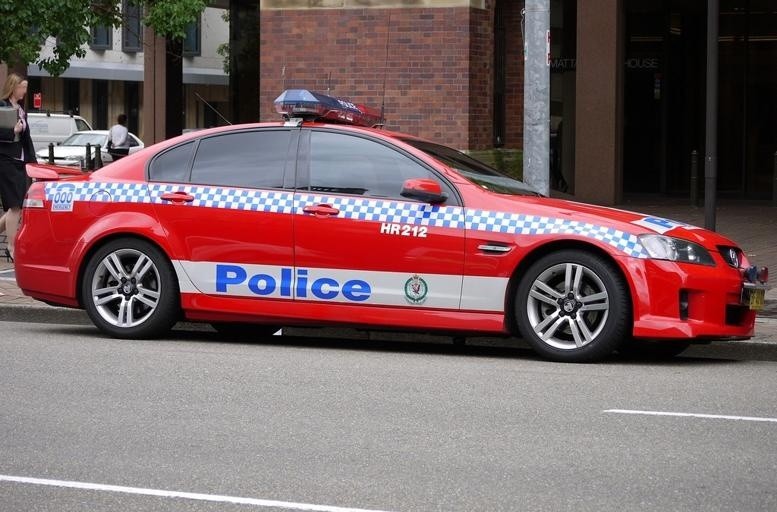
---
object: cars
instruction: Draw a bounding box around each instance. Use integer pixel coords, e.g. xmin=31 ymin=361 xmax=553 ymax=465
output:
xmin=12 ymin=119 xmax=768 ymax=363
xmin=26 ymin=112 xmax=145 ymax=168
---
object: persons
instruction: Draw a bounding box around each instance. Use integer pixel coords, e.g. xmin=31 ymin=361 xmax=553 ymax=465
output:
xmin=104 ymin=113 xmax=131 ymax=163
xmin=0 ymin=72 xmax=37 ymax=264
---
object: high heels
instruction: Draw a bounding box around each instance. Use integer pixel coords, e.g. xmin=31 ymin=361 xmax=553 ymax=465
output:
xmin=5 ymin=248 xmax=15 ymax=263
